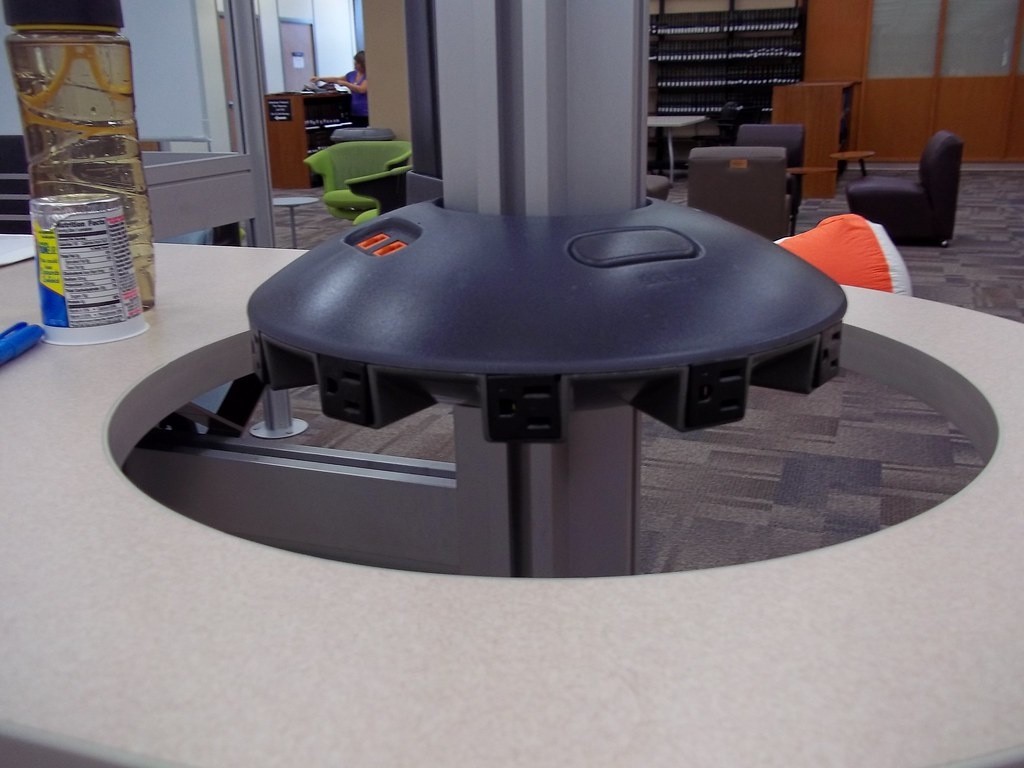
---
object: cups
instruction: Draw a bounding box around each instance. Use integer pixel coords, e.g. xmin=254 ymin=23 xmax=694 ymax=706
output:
xmin=4 ymin=0 xmax=156 ymax=313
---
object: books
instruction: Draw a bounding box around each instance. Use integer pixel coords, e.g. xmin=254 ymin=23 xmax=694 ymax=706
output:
xmin=649 ymin=7 xmax=807 ymax=118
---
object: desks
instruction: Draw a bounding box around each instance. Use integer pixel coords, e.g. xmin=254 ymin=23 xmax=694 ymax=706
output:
xmin=647 ymin=116 xmax=705 ymax=185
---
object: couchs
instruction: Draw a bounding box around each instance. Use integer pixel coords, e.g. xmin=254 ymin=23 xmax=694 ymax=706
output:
xmin=686 ymin=124 xmax=806 ymax=241
xmin=845 ymin=130 xmax=964 ymax=247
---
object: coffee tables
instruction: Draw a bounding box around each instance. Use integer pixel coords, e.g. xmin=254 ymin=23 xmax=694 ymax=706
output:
xmin=273 ymin=197 xmax=319 ymax=248
xmin=829 ymin=151 xmax=876 ymax=175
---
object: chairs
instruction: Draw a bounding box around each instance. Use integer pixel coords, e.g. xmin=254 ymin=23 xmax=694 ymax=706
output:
xmin=303 ymin=141 xmax=413 ymax=226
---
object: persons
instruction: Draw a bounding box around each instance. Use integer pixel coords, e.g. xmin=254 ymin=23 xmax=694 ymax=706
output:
xmin=309 ymin=50 xmax=369 ymax=128
